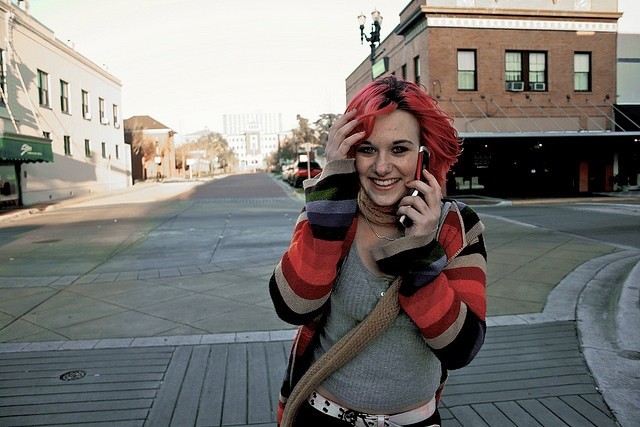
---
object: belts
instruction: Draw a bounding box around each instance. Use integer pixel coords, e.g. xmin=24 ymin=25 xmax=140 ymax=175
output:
xmin=307 ymin=390 xmax=435 ymax=427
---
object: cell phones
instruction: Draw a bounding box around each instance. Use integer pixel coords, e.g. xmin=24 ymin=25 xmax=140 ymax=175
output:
xmin=400 ymin=146 xmax=431 ymax=228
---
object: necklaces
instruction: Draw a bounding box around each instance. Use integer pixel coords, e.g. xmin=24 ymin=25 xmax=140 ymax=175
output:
xmin=366 ymin=216 xmax=404 ymax=241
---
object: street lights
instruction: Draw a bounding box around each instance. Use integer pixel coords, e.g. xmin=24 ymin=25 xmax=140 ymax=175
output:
xmin=358 ymin=8 xmax=382 ymax=83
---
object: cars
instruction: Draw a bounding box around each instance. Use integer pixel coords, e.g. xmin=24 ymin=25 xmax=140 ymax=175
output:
xmin=282 ymin=166 xmax=297 ymax=181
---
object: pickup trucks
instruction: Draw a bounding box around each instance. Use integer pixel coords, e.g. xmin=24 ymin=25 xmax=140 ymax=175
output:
xmin=288 ymin=161 xmax=322 ymax=188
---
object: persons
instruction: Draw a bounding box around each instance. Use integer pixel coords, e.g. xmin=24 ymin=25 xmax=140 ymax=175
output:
xmin=267 ymin=73 xmax=489 ymax=427
